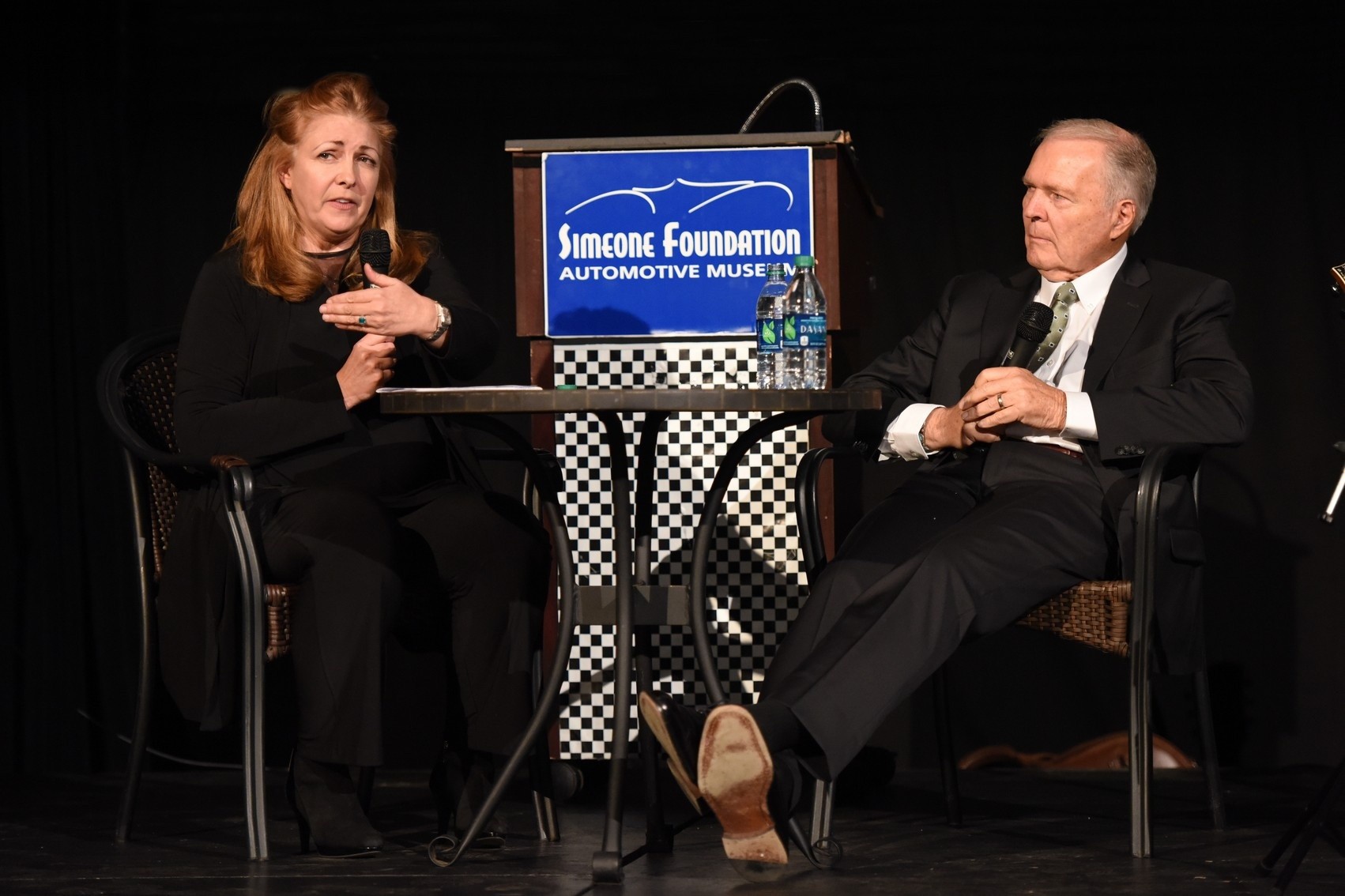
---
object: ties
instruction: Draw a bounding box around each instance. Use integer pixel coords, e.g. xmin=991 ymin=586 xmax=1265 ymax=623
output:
xmin=1024 ymin=282 xmax=1079 ymax=376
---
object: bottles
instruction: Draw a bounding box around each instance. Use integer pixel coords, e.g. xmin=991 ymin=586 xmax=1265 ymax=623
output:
xmin=784 ymin=255 xmax=826 ymax=390
xmin=756 ymin=264 xmax=792 ymax=390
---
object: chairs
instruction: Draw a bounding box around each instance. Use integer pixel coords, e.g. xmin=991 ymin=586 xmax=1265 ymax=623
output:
xmin=797 ymin=436 xmax=1252 ymax=870
xmin=101 ymin=321 xmax=563 ymax=864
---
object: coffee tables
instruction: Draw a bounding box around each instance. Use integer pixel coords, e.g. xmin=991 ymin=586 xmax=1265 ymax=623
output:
xmin=347 ymin=389 xmax=881 ymax=876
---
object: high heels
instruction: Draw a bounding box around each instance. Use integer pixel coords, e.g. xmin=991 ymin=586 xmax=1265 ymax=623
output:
xmin=285 ymin=745 xmax=384 ymax=859
xmin=430 ymin=745 xmax=501 ymax=842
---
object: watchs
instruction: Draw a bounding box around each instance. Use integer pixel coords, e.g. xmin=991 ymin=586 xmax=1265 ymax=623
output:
xmin=422 ymin=300 xmax=452 ymax=343
xmin=918 ymin=420 xmax=936 ymax=453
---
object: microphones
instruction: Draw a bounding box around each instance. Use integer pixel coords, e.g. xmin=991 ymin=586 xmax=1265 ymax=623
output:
xmin=358 ymin=228 xmax=393 ymax=290
xmin=999 ymin=301 xmax=1053 ymax=369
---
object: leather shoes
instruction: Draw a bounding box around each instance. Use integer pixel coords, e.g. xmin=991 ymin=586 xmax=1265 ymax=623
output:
xmin=636 ymin=690 xmax=795 ymax=864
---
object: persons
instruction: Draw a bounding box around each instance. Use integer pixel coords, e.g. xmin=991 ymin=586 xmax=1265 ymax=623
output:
xmin=630 ymin=114 xmax=1254 ymax=879
xmin=166 ymin=67 xmax=566 ymax=868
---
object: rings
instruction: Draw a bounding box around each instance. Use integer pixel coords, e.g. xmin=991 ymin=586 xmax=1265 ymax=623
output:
xmin=997 ymin=393 xmax=1005 ymax=410
xmin=358 ymin=315 xmax=367 ymax=327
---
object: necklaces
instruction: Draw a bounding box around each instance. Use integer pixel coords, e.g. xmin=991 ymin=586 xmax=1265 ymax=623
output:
xmin=317 ymin=268 xmax=339 ymax=286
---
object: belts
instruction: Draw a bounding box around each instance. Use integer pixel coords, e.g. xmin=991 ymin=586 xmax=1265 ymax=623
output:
xmin=1027 ymin=442 xmax=1091 ymax=465
